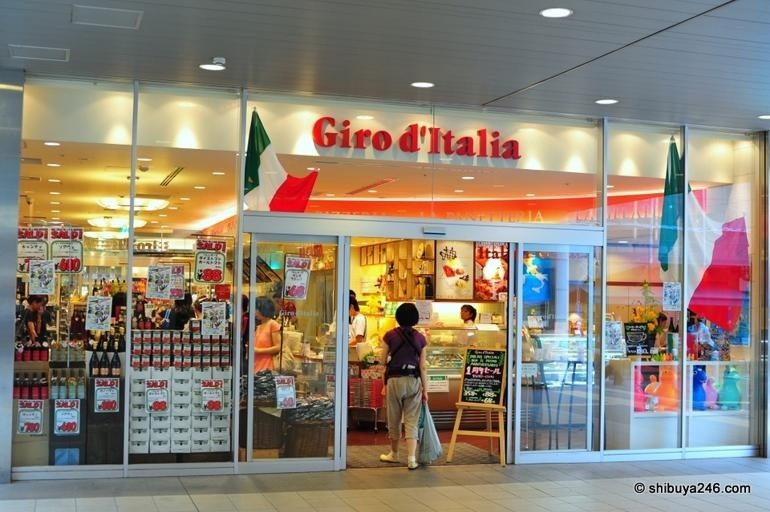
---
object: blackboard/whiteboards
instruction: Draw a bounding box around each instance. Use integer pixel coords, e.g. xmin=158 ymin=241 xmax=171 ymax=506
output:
xmin=624 ymin=323 xmax=652 ymax=359
xmin=459 ymin=346 xmax=507 ymax=407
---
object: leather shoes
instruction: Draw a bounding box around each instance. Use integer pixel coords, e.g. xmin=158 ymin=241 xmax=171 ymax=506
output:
xmin=408 ymin=462 xmax=420 ymax=470
xmin=380 ymin=454 xmax=400 ymax=464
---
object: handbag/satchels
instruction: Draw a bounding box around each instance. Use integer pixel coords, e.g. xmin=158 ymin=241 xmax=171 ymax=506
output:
xmin=160 ymin=308 xmax=171 ymax=330
xmin=14 ymin=310 xmax=30 ymax=340
xmin=271 ymin=347 xmax=297 ymax=376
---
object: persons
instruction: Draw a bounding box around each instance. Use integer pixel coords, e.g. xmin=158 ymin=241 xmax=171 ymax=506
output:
xmin=379 ymin=303 xmax=429 ymax=470
xmin=16 ymin=292 xmax=298 ymax=377
xmin=456 ymin=305 xmax=478 ymax=345
xmin=349 ymin=297 xmax=367 ymax=345
xmin=329 ymin=290 xmax=356 ymax=338
xmin=646 ymin=311 xmax=715 ymax=358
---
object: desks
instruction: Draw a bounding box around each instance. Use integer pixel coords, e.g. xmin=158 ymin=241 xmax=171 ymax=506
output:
xmin=521 ymin=360 xmax=587 ymax=449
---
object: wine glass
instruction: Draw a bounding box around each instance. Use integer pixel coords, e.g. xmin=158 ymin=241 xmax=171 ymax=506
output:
xmin=482 ymin=267 xmax=506 ymax=301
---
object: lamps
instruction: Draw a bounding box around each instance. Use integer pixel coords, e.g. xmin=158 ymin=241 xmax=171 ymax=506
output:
xmin=83 ymin=231 xmax=129 ymax=239
xmin=88 ymin=218 xmax=147 ymax=228
xmin=95 ymin=196 xmax=169 ymax=211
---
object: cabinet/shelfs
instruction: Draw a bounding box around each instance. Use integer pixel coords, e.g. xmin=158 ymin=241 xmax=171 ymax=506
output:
xmin=359 ymin=239 xmax=435 ymax=301
xmin=12 ymin=350 xmax=124 ymax=465
xmin=605 ymin=359 xmax=750 ymax=450
xmin=424 ymin=327 xmax=479 ymax=372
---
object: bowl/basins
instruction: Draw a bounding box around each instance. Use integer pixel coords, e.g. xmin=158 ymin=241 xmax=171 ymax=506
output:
xmin=349 ymin=348 xmax=387 ymax=380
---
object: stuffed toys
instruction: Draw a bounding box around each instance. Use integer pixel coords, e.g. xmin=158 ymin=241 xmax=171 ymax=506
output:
xmin=634 ymin=364 xmax=742 ymax=412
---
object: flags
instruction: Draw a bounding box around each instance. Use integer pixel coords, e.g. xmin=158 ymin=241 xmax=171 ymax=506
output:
xmin=243 ymin=111 xmax=318 ymax=213
xmin=658 ymin=141 xmax=750 ymax=333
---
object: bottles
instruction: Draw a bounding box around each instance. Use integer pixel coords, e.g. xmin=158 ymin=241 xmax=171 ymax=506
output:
xmin=111 ymin=340 xmax=121 ymax=377
xmin=88 ymin=313 xmax=127 ymax=352
xmin=89 ymin=340 xmax=101 ymax=377
xmin=13 ymin=372 xmax=21 ymax=399
xmin=132 ymin=279 xmax=175 ymax=330
xmin=76 ymin=369 xmax=87 ymax=399
xmin=93 ymin=276 xmax=126 ymax=295
xmin=21 ymin=372 xmax=32 ymax=399
xmin=67 ymin=368 xmax=78 ymax=399
xmin=188 ymin=282 xmax=233 ymax=320
xmin=276 ymin=343 xmax=336 ymax=401
xmin=384 ymin=273 xmax=433 ymax=300
xmin=50 ymin=369 xmax=59 ymax=400
xmin=100 ymin=340 xmax=110 ymax=377
xmin=49 ymin=334 xmax=86 ymax=368
xmin=32 ymin=338 xmax=40 ymax=361
xmin=59 ymin=369 xmax=69 ymax=399
xmin=30 ymin=371 xmax=40 ymax=399
xmin=40 ymin=336 xmax=50 ymax=361
xmin=39 ymin=372 xmax=49 ymax=400
xmin=15 ymin=334 xmax=23 ymax=362
xmin=23 ymin=336 xmax=32 ymax=361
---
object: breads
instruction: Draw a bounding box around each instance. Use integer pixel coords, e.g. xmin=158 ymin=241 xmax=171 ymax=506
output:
xmin=429 ymin=334 xmax=457 ymax=346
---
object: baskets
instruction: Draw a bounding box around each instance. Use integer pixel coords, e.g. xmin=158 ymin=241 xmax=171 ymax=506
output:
xmin=278 ymin=418 xmax=330 ymax=459
xmin=240 ymin=408 xmax=283 ymax=450
